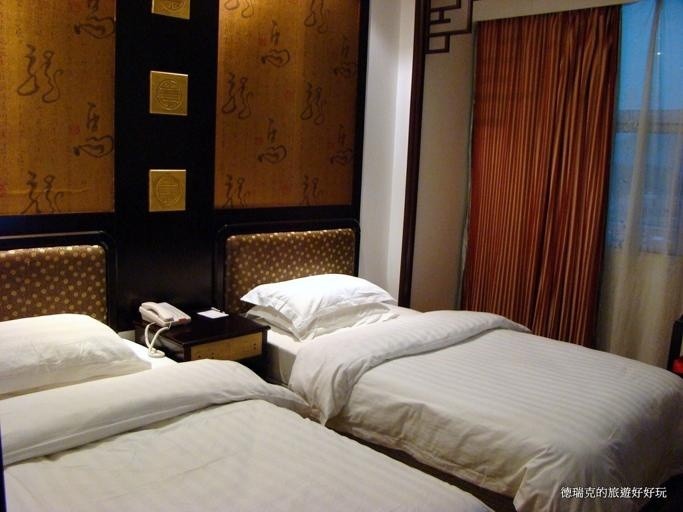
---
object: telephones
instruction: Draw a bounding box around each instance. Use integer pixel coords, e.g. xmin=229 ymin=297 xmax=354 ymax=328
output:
xmin=138 ymin=301 xmax=191 ymax=327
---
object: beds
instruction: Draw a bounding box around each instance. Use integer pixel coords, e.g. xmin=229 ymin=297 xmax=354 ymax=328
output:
xmin=0 ymin=229 xmax=495 ymax=512
xmin=222 ymin=204 xmax=683 ymax=512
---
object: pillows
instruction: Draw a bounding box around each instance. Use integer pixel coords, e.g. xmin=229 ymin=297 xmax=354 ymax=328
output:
xmin=0 ymin=314 xmax=150 ymax=396
xmin=245 ymin=305 xmax=401 ymax=343
xmin=240 ymin=273 xmax=397 ymax=331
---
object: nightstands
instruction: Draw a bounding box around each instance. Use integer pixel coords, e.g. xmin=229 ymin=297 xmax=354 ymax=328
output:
xmin=132 ymin=305 xmax=270 ymax=382
